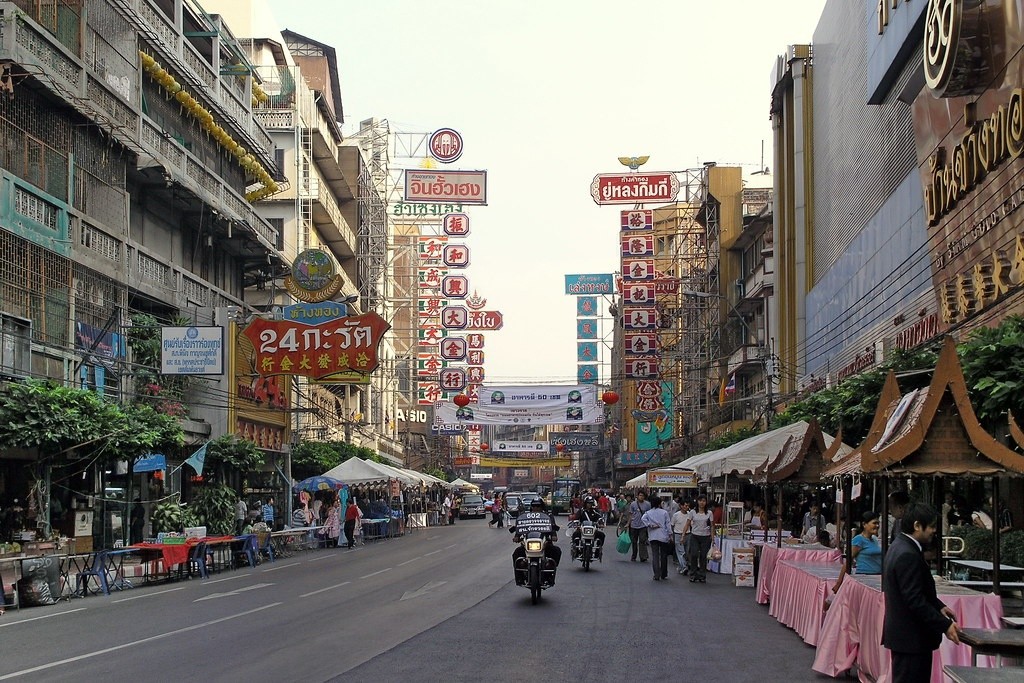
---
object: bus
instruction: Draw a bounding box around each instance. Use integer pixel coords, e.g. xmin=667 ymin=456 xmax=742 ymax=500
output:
xmin=552 ymin=477 xmax=582 ymax=516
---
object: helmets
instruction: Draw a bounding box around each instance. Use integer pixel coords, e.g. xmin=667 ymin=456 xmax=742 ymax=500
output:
xmin=531 ymin=496 xmax=545 ymax=507
xmin=583 ymin=495 xmax=595 ymax=503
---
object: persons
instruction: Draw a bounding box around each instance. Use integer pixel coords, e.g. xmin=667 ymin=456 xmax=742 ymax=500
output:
xmin=131 ymin=495 xmax=145 ymax=545
xmin=710 ymin=499 xmax=726 ymax=524
xmin=234 ymin=495 xmax=274 ymax=534
xmin=293 ymin=498 xmax=361 ymax=549
xmin=441 ymin=493 xmax=458 ymax=525
xmin=940 ymin=489 xmax=1013 ymax=539
xmin=887 ymin=491 xmax=909 ymax=545
xmin=623 ymin=491 xmax=715 ymax=583
xmin=570 ymin=488 xmax=635 ymax=524
xmin=743 ymin=493 xmax=776 ymax=529
xmin=798 ymin=502 xmax=826 ymax=539
xmin=881 ymin=502 xmax=960 ymax=683
xmin=569 ymin=495 xmax=605 ymax=558
xmin=489 ymin=491 xmax=512 ymax=529
xmin=513 ymin=497 xmax=558 ymax=542
xmin=831 ymin=512 xmax=881 ymax=594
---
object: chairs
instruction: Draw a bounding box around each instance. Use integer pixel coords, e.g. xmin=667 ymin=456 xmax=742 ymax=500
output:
xmin=73 ymin=549 xmax=110 ymax=597
xmin=257 ymin=530 xmax=274 ymax=563
xmin=151 ymin=556 xmax=172 ymax=580
xmin=230 ymin=535 xmax=257 ymax=570
xmin=176 ymin=539 xmax=210 ymax=581
xmin=192 ymin=549 xmax=216 ymax=574
xmin=373 ymin=521 xmax=389 ymax=543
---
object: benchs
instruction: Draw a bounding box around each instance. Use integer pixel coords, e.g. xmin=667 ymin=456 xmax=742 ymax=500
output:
xmin=952 ymin=580 xmax=1024 ymax=598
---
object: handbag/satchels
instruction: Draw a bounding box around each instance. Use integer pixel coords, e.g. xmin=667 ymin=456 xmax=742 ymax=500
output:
xmin=707 ymin=543 xmax=722 ymax=563
xmin=616 ymin=529 xmax=631 ymax=554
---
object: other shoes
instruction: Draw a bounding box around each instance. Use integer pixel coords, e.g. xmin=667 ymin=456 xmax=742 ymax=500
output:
xmin=689 ymin=576 xmax=696 ymax=582
xmin=653 ymin=575 xmax=660 ymax=580
xmin=661 ymin=570 xmax=668 ymax=579
xmin=680 ymin=568 xmax=688 ymax=576
xmin=488 ymin=522 xmax=491 ymax=528
xmin=698 ymin=578 xmax=706 ymax=583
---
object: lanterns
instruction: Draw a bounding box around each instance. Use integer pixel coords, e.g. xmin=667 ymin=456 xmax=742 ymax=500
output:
xmin=601 ymin=390 xmax=619 ymax=406
xmin=454 ymin=395 xmax=470 ymax=408
xmin=480 ymin=443 xmax=489 ymax=451
xmin=555 ymin=444 xmax=564 ymax=453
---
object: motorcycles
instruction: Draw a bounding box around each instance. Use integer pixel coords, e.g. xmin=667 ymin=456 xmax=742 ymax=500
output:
xmin=567 ymin=518 xmax=606 ymax=572
xmin=509 ymin=508 xmax=560 ymax=606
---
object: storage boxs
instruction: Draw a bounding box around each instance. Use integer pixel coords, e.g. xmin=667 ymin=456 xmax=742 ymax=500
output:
xmin=732 ymin=564 xmax=754 ymax=576
xmin=733 ymin=548 xmax=753 ymax=553
xmin=733 ymin=576 xmax=754 ymax=587
xmin=732 ymin=553 xmax=753 ymax=564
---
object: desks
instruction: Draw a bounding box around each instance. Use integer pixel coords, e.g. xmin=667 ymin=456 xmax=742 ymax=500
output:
xmin=0 ymin=517 xmax=406 ymax=613
xmin=429 ymin=511 xmax=439 ymax=524
xmin=710 ymin=523 xmax=1024 ymax=683
xmin=407 ymin=513 xmax=429 ymax=528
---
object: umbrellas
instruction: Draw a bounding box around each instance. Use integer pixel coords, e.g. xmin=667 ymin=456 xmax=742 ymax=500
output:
xmin=292 ymin=476 xmax=348 ymax=509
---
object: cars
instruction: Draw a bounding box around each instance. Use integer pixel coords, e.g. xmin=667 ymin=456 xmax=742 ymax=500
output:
xmin=459 ymin=495 xmax=487 ymax=520
xmin=506 ymin=496 xmax=525 ymax=518
xmin=506 ymin=492 xmax=544 ymax=512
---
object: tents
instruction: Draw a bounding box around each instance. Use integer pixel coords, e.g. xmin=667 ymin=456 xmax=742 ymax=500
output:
xmin=625 ymin=420 xmax=855 ymax=575
xmin=320 ymin=456 xmax=479 ymax=539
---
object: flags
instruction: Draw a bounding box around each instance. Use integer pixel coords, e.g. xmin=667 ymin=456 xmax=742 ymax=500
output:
xmin=724 ymin=373 xmax=735 ymax=396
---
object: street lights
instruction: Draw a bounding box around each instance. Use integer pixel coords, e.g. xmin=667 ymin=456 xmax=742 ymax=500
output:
xmin=682 ymin=290 xmax=773 ymax=419
